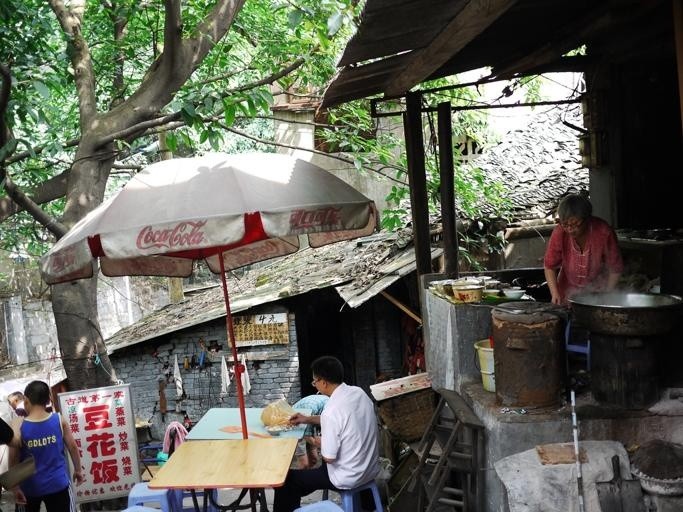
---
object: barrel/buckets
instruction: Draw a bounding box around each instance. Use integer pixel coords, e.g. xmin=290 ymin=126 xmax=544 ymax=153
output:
xmin=473 ymin=339 xmax=498 ymax=393
xmin=630 ymin=443 xmax=683 ymax=512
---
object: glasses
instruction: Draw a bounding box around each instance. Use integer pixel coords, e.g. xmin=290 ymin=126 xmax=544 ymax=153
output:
xmin=311 ymin=378 xmax=321 ymax=387
xmin=560 ymin=220 xmax=585 ymax=229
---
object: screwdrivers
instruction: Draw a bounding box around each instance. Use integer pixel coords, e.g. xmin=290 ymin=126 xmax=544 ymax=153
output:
xmin=184 ymin=337 xmax=195 ymax=369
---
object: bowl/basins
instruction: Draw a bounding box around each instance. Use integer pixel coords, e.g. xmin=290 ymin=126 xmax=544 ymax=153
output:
xmin=432 ymin=274 xmax=501 ymax=301
xmin=264 ymin=425 xmax=286 ymax=436
xmin=504 ymin=289 xmax=526 ymax=300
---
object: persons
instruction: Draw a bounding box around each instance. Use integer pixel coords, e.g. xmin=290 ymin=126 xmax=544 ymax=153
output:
xmin=0 ymin=391 xmax=26 ymax=417
xmin=289 ymin=395 xmax=328 ymax=468
xmin=544 ymin=191 xmax=627 ymax=309
xmin=273 ymin=356 xmax=380 ymax=512
xmin=9 ymin=382 xmax=84 ymax=512
xmin=0 ymin=418 xmax=22 ymax=451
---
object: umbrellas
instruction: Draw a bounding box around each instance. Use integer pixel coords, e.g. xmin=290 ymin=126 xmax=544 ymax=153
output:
xmin=35 ymin=153 xmax=381 ymax=439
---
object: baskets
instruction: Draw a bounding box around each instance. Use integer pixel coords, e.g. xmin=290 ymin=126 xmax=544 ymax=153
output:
xmin=378 ymin=391 xmax=436 ymax=438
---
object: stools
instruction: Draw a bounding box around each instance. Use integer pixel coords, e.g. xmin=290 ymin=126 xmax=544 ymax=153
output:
xmin=293 ymin=479 xmax=382 ymax=511
xmin=119 ymin=481 xmax=176 ymax=511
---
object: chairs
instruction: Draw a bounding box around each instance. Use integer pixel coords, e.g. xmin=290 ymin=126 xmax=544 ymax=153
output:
xmin=139 ymin=426 xmax=175 ymax=481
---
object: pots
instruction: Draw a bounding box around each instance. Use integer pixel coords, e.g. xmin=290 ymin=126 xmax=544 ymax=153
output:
xmin=568 ymin=289 xmax=681 ymax=335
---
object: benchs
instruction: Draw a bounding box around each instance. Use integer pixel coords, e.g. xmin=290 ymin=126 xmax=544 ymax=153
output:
xmin=384 ymin=389 xmax=483 ymax=511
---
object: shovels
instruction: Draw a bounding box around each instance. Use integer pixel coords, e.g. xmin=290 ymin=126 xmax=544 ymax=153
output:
xmin=595 ymin=455 xmax=646 ymax=512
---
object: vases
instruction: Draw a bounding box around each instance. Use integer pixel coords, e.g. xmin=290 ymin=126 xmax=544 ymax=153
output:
xmin=630 ymin=468 xmax=683 ymax=495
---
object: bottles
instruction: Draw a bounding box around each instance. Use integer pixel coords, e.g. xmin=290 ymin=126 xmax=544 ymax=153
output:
xmin=182 ymin=411 xmax=192 ymax=433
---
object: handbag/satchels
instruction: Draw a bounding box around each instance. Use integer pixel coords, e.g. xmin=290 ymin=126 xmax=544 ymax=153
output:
xmin=1 ymin=454 xmax=36 ymax=491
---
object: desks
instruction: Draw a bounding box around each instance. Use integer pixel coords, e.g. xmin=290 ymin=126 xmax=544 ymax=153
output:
xmin=149 ymin=407 xmax=307 ymax=511
